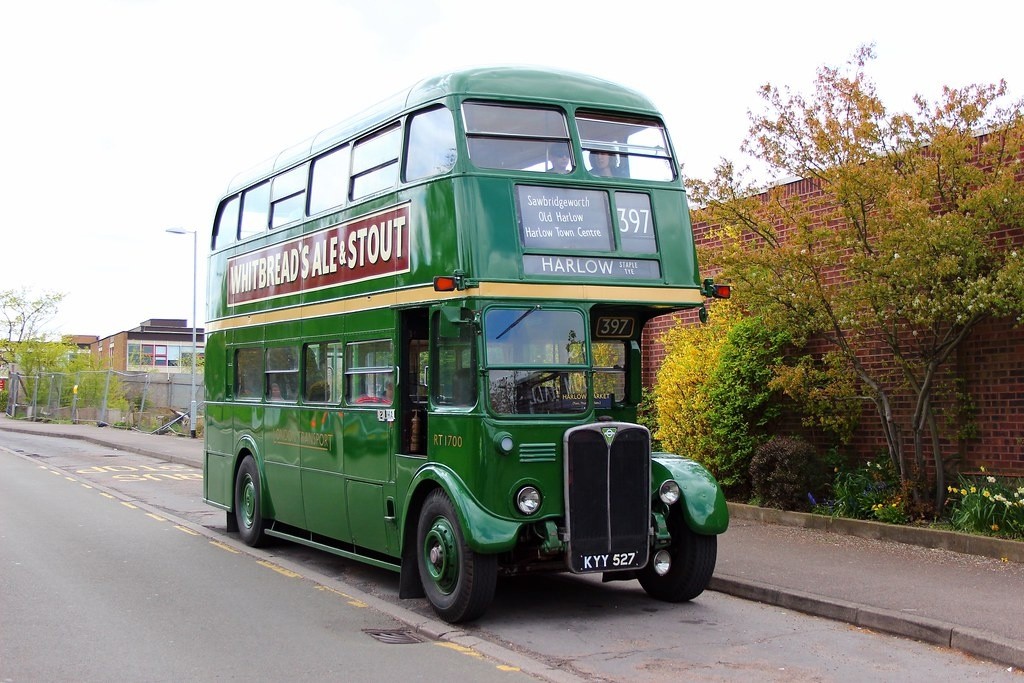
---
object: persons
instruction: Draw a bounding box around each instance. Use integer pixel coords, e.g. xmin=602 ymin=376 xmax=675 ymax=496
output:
xmin=546 ymin=143 xmax=571 ymax=174
xmin=588 ymin=151 xmax=629 ymax=178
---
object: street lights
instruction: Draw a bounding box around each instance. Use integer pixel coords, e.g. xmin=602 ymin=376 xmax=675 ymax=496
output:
xmin=165 ymin=226 xmax=199 ymax=438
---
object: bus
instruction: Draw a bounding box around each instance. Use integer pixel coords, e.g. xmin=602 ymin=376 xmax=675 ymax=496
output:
xmin=202 ymin=64 xmax=732 ymax=624
